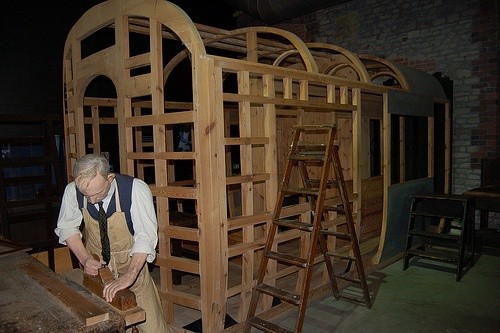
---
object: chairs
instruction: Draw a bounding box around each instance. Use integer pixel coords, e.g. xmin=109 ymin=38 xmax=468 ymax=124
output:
xmin=464 ymin=157 xmax=500 ymax=255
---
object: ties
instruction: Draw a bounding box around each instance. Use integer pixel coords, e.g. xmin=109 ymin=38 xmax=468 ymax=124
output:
xmin=97 ymin=200 xmax=111 ymax=265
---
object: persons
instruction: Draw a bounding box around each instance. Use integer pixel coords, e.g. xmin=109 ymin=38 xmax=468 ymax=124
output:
xmin=53 ymin=154 xmax=167 ymax=333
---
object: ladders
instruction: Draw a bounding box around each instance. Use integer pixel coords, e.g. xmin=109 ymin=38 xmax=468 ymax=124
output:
xmin=242 ymin=123 xmax=372 ymax=333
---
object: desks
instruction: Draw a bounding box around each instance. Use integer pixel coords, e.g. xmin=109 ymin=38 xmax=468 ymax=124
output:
xmin=0 ymin=253 xmax=124 ymax=333
